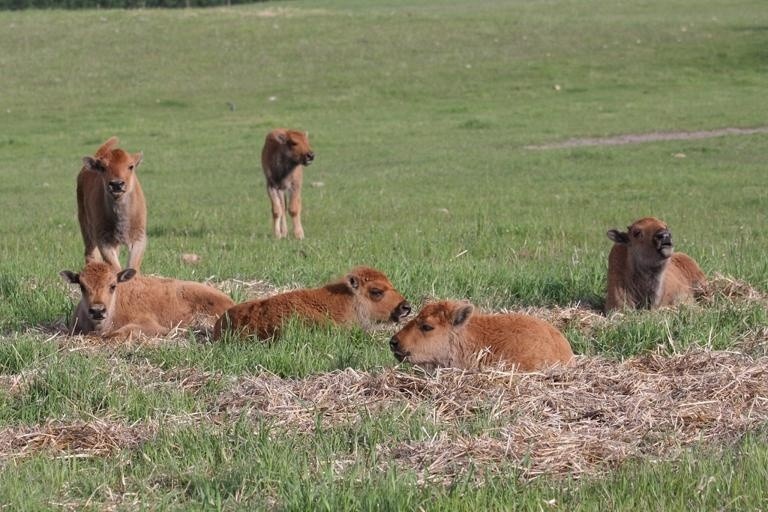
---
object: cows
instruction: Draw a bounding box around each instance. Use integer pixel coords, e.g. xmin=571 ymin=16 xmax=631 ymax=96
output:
xmin=388 ymin=300 xmax=578 ymax=375
xmin=261 ymin=128 xmax=315 ymax=240
xmin=213 ymin=267 xmax=411 ymax=351
xmin=58 ymin=261 xmax=238 ymax=342
xmin=603 ymin=217 xmax=709 ymax=320
xmin=76 ymin=136 xmax=147 ymax=274
